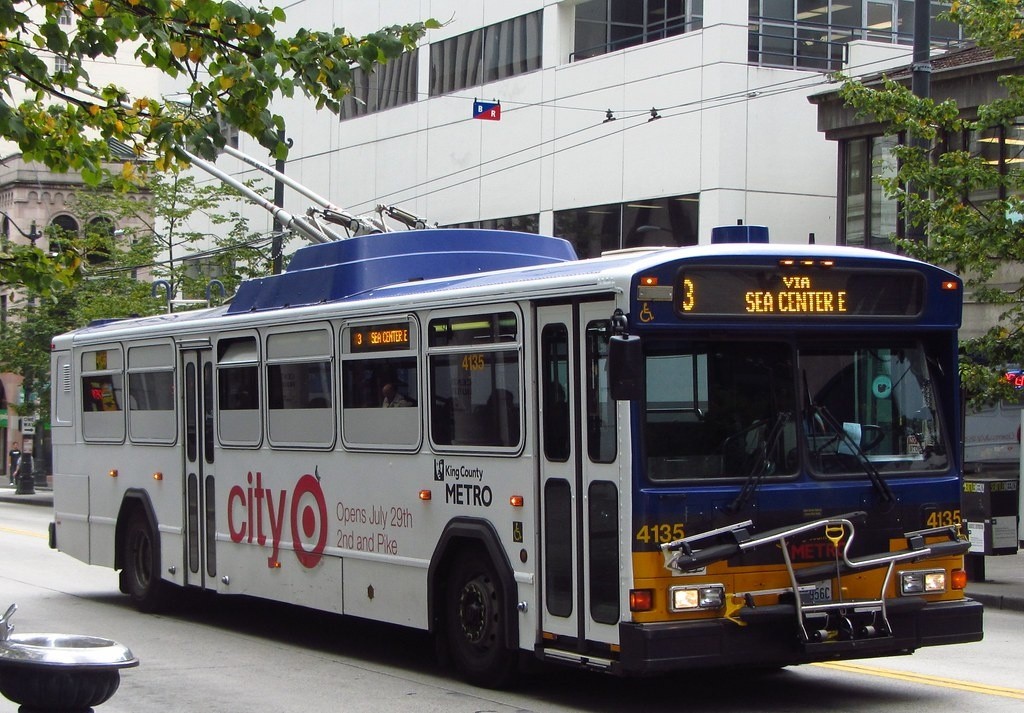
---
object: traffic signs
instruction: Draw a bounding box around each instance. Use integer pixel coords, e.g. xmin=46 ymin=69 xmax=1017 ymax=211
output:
xmin=22 ymin=416 xmax=36 ymax=435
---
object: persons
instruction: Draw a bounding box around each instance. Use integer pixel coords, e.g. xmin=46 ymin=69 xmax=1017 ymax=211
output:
xmin=745 ymin=379 xmax=825 ymax=474
xmin=382 ymin=384 xmax=409 ymax=408
xmin=12 ymin=448 xmax=35 ymax=495
xmin=8 ymin=441 xmax=21 ymax=485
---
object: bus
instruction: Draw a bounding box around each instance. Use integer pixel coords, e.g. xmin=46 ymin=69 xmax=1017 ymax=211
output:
xmin=43 ymin=85 xmax=988 ymax=692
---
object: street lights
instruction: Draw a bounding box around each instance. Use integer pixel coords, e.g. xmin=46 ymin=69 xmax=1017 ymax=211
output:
xmin=170 ymin=99 xmax=294 ymax=276
xmin=113 ymin=227 xmax=139 ymax=282
